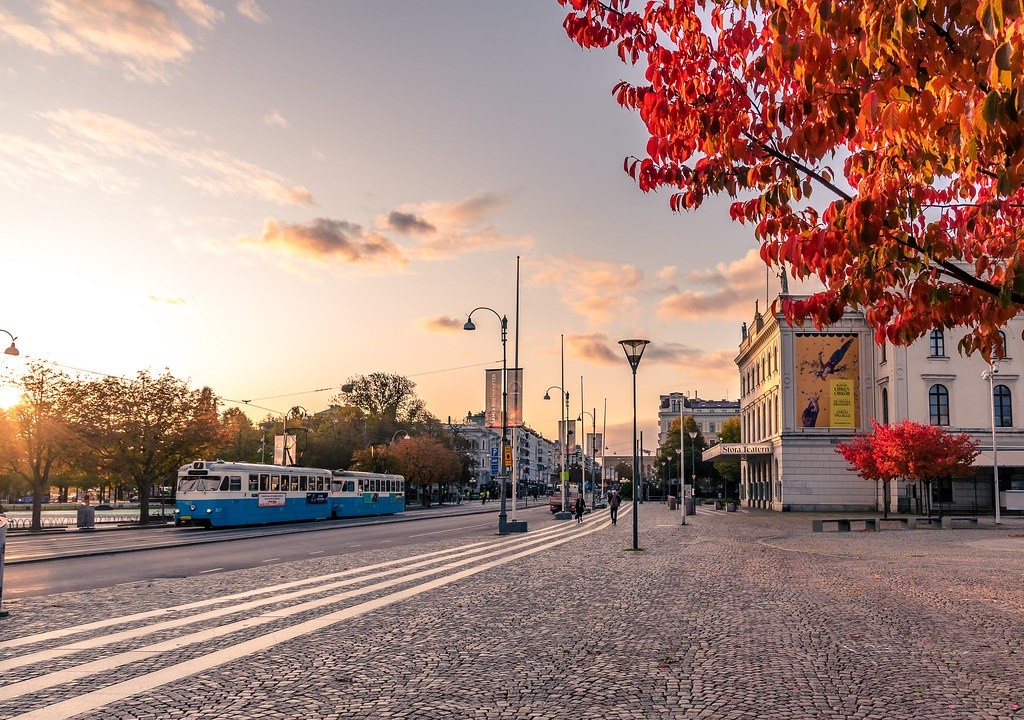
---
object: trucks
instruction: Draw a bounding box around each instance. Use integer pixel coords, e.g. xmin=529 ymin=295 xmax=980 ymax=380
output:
xmin=549 ymin=484 xmax=579 ymax=513
xmin=545 ymin=484 xmax=555 ymax=495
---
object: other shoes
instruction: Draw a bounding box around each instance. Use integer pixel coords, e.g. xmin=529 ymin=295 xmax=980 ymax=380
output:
xmin=578 ymin=519 xmax=583 ymax=523
xmin=612 ymin=519 xmax=616 ymax=525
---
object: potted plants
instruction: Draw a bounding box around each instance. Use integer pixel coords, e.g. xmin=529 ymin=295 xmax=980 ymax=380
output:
xmin=725 ymin=498 xmax=736 ymax=512
xmin=713 ymin=497 xmax=722 ymax=509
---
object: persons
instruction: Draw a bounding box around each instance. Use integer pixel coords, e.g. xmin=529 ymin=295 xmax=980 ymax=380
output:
xmin=818 ymin=337 xmax=855 ymax=381
xmin=801 ymin=395 xmax=820 ymax=427
xmin=480 ymin=490 xmax=490 ymax=505
xmin=457 ymin=489 xmax=463 ymax=504
xmin=576 ymin=494 xmax=586 ymax=523
xmin=532 ymin=490 xmax=538 ymax=501
xmin=440 ymin=492 xmax=448 ymax=504
xmin=466 ymin=491 xmax=473 ymax=501
xmin=608 ymin=489 xmax=621 ymax=525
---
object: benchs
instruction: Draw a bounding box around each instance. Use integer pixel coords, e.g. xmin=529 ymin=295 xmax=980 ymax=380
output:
xmin=821 ymin=520 xmax=850 ymax=531
xmin=952 ymin=517 xmax=978 ymax=528
xmin=917 ymin=517 xmax=942 ymax=529
xmin=880 ymin=518 xmax=908 ymax=530
xmin=849 ymin=519 xmax=876 ymax=530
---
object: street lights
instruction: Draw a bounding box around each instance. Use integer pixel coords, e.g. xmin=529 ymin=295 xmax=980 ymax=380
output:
xmin=469 ymin=477 xmax=476 ymax=496
xmin=981 ymin=360 xmax=1000 ymax=523
xmin=667 ymin=456 xmax=673 ymax=496
xmin=689 ymin=431 xmax=698 ymax=515
xmin=618 ymin=339 xmax=651 ymax=549
xmin=661 ymin=461 xmax=667 ymax=503
xmin=282 ymin=406 xmax=309 ymax=466
xmin=577 ymin=412 xmax=596 ymax=510
xmin=674 ymin=447 xmax=682 ymax=510
xmin=463 ymin=307 xmax=509 ymax=536
xmin=544 ymin=386 xmax=570 ymax=513
xmin=392 ymin=431 xmax=410 ymax=442
xmin=258 ymin=438 xmax=266 ymax=463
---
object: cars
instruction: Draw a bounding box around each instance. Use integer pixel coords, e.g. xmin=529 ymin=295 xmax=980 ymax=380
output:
xmin=51 ymin=491 xmax=60 ymax=500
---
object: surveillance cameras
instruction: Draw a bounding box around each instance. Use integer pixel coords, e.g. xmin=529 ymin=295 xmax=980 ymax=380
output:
xmin=991 ymin=359 xmax=995 ymax=364
xmin=993 ymin=367 xmax=998 ymax=373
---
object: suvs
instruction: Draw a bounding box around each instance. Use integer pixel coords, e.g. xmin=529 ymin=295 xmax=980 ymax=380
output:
xmin=69 ymin=492 xmax=85 ymax=499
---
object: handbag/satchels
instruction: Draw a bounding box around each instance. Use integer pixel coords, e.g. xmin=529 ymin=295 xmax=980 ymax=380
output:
xmin=575 ymin=514 xmax=578 ymax=519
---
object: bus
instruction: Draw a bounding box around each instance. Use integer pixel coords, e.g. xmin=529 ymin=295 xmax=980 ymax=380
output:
xmin=175 ymin=460 xmax=406 ymax=530
xmin=16 ymin=479 xmax=50 ymax=503
